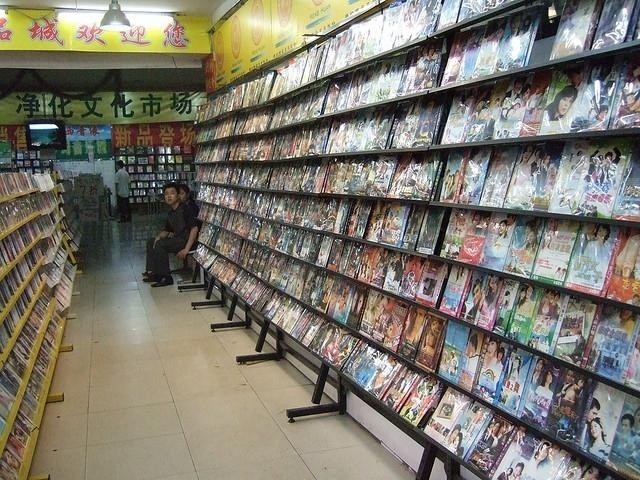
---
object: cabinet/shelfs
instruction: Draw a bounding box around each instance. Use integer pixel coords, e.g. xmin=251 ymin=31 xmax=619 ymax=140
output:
xmin=0 ymin=150 xmax=84 ymax=480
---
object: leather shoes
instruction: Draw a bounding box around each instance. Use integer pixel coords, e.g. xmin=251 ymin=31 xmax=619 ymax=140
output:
xmin=142 ymin=269 xmax=173 ymax=287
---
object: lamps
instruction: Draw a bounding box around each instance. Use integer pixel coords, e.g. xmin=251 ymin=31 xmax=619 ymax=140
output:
xmin=100 ymin=0 xmax=130 ymax=29
xmin=55 ymin=10 xmax=176 ymax=25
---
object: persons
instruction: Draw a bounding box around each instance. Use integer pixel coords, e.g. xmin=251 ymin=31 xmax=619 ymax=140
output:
xmin=142 ymin=181 xmax=195 ymax=294
xmin=114 ymin=160 xmax=132 ymax=224
